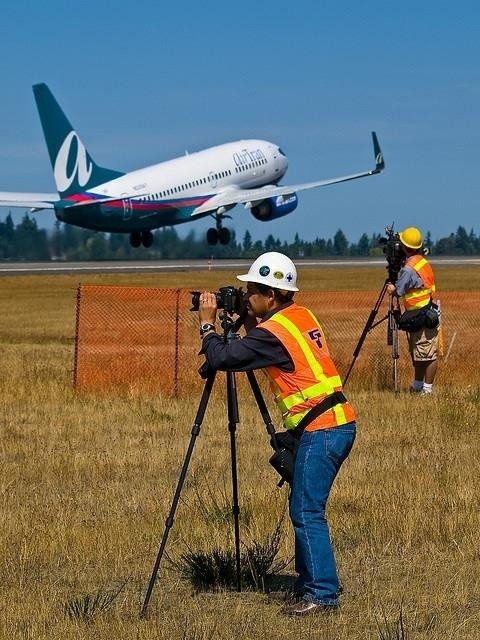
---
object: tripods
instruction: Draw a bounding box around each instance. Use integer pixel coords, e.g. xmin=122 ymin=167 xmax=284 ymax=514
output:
xmin=138 ymin=317 xmax=279 ymax=617
xmin=342 ymin=265 xmax=410 ymax=400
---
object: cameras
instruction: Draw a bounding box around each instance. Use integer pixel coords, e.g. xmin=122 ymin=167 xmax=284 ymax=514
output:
xmin=379 ymin=237 xmax=407 ymax=265
xmin=189 ymin=286 xmax=249 ymax=314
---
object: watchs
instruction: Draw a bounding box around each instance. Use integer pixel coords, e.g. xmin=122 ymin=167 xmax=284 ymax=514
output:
xmin=200 ymin=322 xmax=215 ymax=336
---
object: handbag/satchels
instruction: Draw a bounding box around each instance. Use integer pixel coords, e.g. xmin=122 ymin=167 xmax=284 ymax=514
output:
xmin=400 ymin=308 xmax=440 ymax=334
xmin=268 ymin=430 xmax=298 ymax=488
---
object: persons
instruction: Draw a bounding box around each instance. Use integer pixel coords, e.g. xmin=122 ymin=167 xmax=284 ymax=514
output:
xmin=198 ymin=252 xmax=357 ymax=618
xmin=387 ymin=226 xmax=441 ymax=398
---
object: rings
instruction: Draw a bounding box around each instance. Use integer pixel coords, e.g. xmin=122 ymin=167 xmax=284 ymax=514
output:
xmin=203 ymin=298 xmax=208 ymax=301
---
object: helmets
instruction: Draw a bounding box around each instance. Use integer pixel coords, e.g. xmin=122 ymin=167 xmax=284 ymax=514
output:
xmin=236 ymin=251 xmax=301 ymax=293
xmin=399 ymin=226 xmax=424 ymax=250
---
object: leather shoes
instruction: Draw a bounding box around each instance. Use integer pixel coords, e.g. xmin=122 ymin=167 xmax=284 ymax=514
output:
xmin=269 ymin=584 xmax=337 ymax=616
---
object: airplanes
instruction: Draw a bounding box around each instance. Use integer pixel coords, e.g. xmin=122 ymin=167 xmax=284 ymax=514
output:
xmin=0 ymin=83 xmax=385 ymax=248
